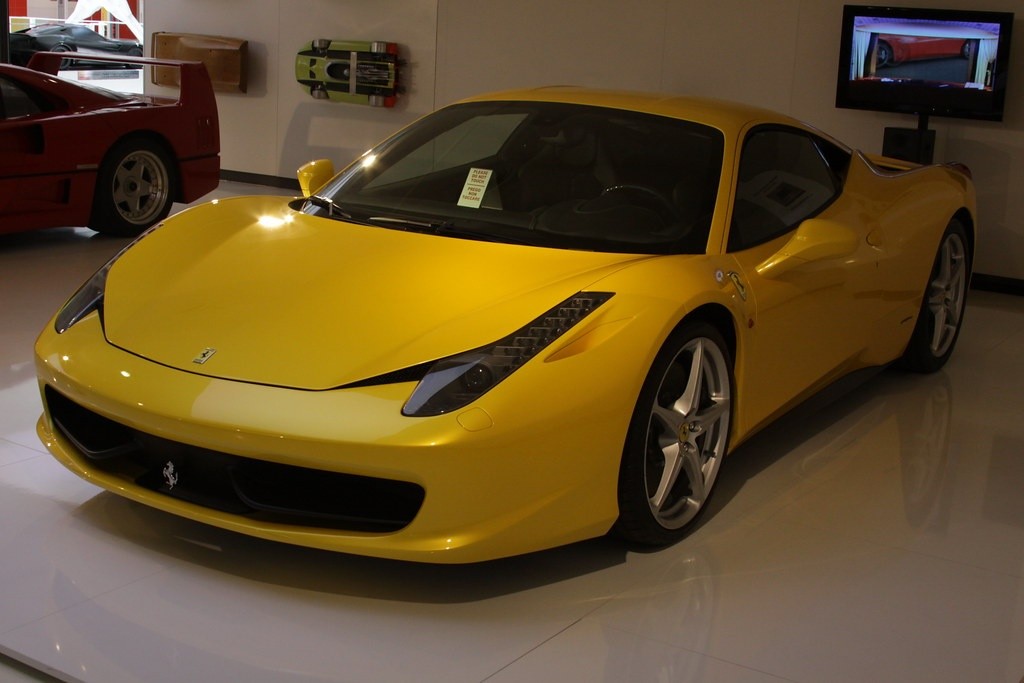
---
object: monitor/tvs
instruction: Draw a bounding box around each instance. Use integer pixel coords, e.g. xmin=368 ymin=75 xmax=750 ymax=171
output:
xmin=834 ymin=4 xmax=1015 ymax=124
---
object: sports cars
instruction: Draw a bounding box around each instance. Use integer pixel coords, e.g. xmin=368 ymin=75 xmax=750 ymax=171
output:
xmin=4 ymin=24 xmax=145 ymax=69
xmin=873 ymin=35 xmax=974 ymax=72
xmin=33 ymin=83 xmax=980 ymax=565
xmin=0 ymin=49 xmax=225 ymax=236
